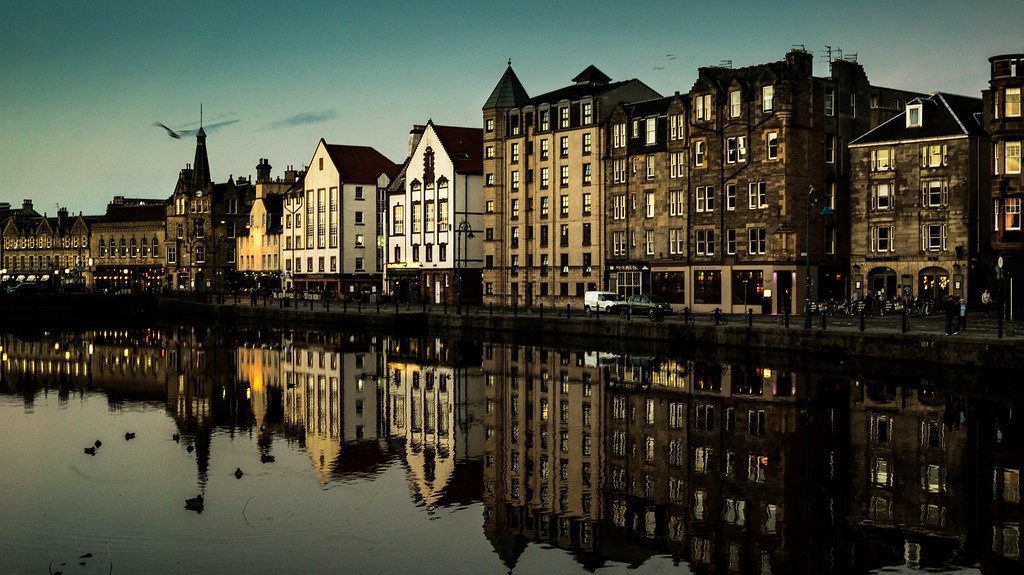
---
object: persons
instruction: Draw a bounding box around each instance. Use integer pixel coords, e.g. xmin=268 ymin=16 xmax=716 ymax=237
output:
xmin=945 ymin=295 xmax=967 ymax=335
xmin=824 ymin=289 xmax=928 ymax=317
xmin=982 ymin=288 xmax=990 ymax=316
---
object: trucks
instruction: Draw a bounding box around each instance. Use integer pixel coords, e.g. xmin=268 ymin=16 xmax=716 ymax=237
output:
xmin=584 ymin=291 xmax=631 ymax=317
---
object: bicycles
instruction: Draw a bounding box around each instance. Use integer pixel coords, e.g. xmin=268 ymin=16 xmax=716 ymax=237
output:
xmin=648 ymin=305 xmax=666 ymax=323
xmin=681 ymin=308 xmax=696 ymax=323
xmin=711 ymin=309 xmax=729 ymax=325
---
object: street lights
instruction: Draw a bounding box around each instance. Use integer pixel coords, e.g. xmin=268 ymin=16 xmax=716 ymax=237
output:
xmin=742 ymin=279 xmax=750 ymax=322
xmin=212 ymin=214 xmax=228 ymax=304
xmin=456 ymin=221 xmax=476 ymax=316
xmin=803 ymin=187 xmax=833 ymax=328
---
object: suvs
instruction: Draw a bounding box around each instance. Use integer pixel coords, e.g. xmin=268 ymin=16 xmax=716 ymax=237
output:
xmin=628 ymin=294 xmax=673 ymax=316
